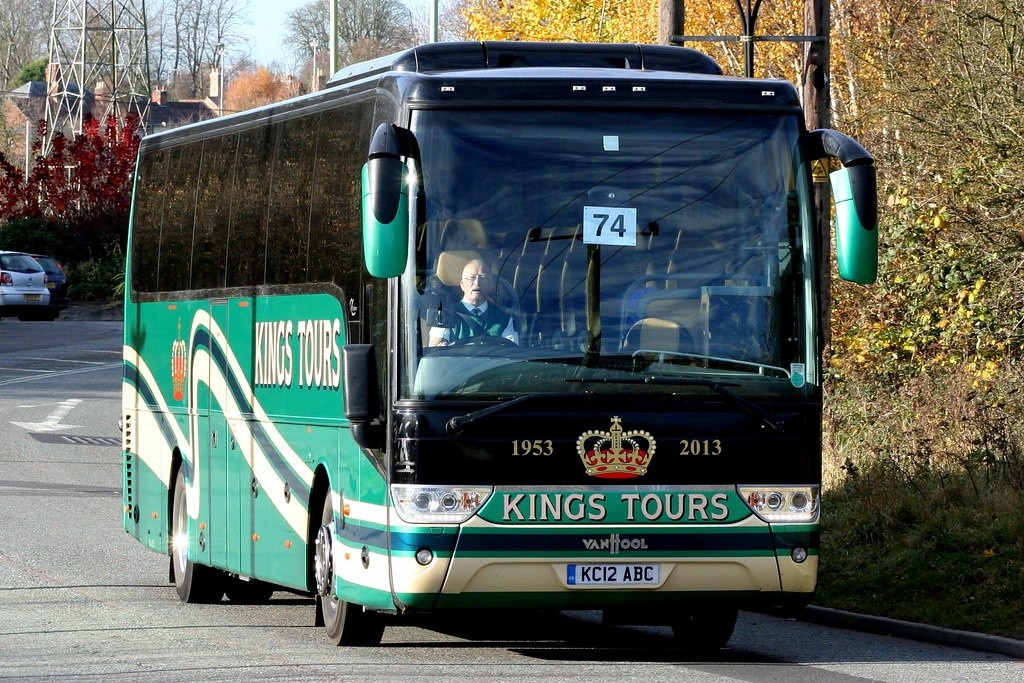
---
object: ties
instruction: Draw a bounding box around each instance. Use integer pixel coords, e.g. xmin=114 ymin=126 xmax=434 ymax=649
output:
xmin=472 ymin=309 xmax=479 ymax=317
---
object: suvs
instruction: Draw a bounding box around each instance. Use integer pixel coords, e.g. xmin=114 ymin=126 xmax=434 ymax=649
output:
xmin=27 ymin=253 xmax=67 ymax=321
xmin=0 ymin=248 xmax=51 ymax=323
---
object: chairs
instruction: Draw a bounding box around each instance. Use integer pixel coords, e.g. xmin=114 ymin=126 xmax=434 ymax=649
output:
xmin=416 ymin=219 xmax=735 ymax=363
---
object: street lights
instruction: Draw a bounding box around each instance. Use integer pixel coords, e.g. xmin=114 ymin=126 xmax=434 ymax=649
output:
xmin=215 ymin=42 xmax=226 ymax=117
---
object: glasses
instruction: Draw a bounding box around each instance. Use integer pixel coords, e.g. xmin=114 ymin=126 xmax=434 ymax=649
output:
xmin=463 ymin=276 xmax=492 ymax=281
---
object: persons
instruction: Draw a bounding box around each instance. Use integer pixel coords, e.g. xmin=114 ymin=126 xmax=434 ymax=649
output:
xmin=428 ymin=258 xmax=520 ymax=347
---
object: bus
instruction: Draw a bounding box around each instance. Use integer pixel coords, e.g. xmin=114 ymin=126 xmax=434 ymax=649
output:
xmin=122 ymin=40 xmax=879 ymax=652
